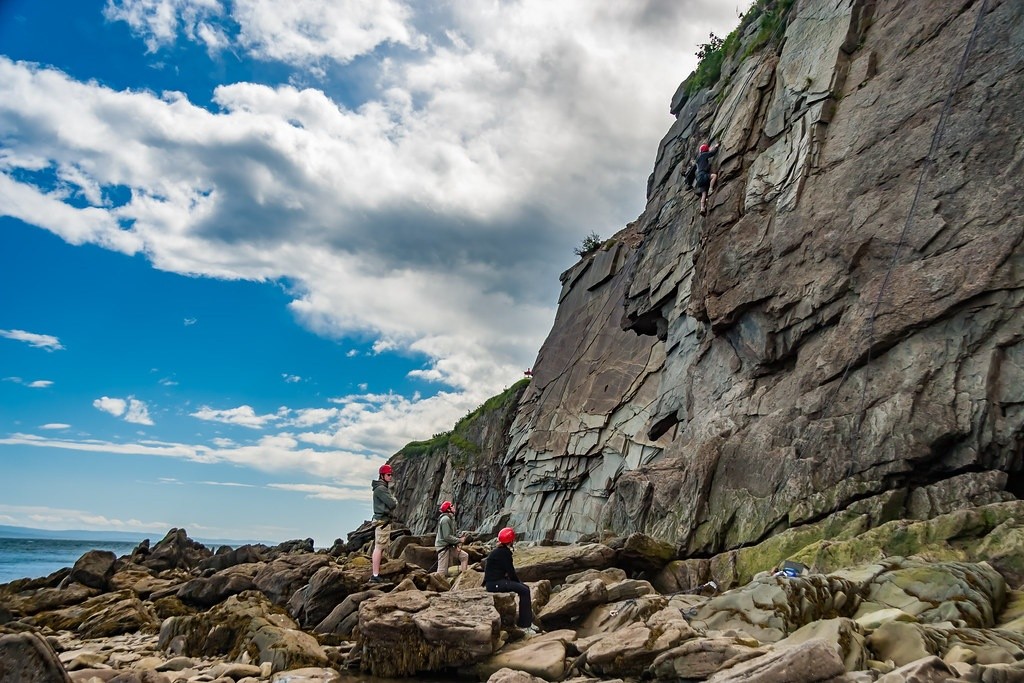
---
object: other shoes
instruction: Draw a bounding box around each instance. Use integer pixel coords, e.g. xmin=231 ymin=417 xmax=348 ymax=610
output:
xmin=515 ymin=622 xmax=539 ymax=630
xmin=516 ymin=626 xmax=536 ymax=635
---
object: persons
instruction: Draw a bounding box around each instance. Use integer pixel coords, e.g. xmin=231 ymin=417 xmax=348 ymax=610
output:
xmin=695 ymin=143 xmax=719 ymax=214
xmin=434 ymin=501 xmax=469 ymax=578
xmin=371 ymin=465 xmax=406 ymax=582
xmin=482 ymin=528 xmax=538 ymax=636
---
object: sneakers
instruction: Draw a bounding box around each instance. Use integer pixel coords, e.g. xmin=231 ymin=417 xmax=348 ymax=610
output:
xmin=369 ymin=573 xmax=390 ymax=583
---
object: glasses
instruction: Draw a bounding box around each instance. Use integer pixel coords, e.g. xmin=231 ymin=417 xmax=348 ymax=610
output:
xmin=385 ymin=473 xmax=394 ymax=477
xmin=450 ymin=504 xmax=454 ymax=507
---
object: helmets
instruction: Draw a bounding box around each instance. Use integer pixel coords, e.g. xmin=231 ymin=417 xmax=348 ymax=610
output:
xmin=498 ymin=528 xmax=514 ymax=543
xmin=441 ymin=501 xmax=452 ymax=512
xmin=379 ymin=465 xmax=393 ymax=474
